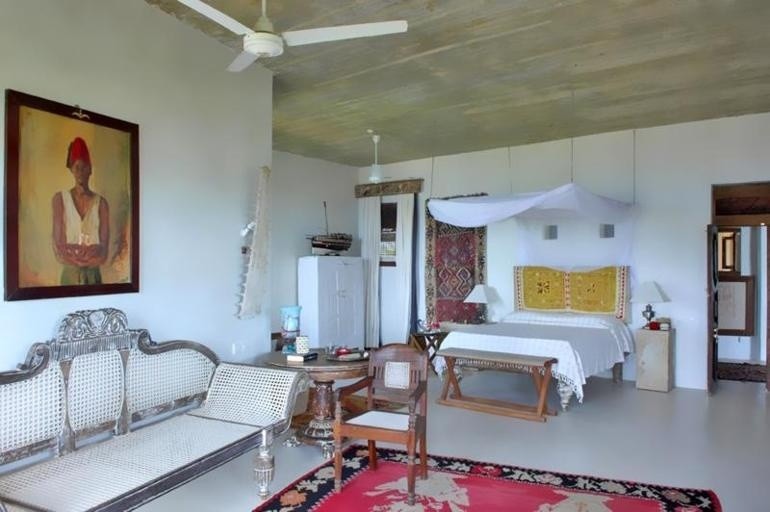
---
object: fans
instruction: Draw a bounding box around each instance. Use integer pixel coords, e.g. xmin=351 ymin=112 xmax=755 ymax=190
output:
xmin=179 ymin=0 xmax=409 ymax=73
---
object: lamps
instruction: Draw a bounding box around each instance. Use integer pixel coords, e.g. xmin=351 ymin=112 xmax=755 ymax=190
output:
xmin=462 ymin=284 xmax=498 ymax=324
xmin=368 ymin=133 xmax=383 ymax=183
xmin=629 ymin=281 xmax=672 ymax=330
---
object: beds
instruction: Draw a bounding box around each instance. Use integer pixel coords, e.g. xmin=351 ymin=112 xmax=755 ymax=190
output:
xmin=437 ymin=264 xmax=633 ymax=413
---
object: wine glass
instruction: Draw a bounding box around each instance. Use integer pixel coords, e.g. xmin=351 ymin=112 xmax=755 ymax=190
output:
xmin=278 ymin=305 xmax=303 ymax=353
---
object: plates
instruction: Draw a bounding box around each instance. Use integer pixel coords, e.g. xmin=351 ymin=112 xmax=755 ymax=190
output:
xmin=327 ymin=349 xmax=370 ymax=362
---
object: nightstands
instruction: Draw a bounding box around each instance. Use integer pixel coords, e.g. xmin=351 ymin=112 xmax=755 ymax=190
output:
xmin=635 ymin=330 xmax=672 ymax=392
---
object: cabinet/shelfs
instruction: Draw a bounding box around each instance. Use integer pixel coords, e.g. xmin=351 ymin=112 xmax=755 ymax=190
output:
xmin=296 ymin=256 xmax=367 ymax=350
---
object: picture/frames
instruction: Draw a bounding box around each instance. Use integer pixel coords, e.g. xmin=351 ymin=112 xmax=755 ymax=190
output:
xmin=4 ymin=89 xmax=141 ymax=300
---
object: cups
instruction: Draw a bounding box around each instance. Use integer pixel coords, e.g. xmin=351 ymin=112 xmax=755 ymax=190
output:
xmin=296 ymin=336 xmax=310 ymax=354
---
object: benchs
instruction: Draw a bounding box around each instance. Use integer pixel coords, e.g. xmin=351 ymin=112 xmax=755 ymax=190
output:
xmin=435 ymin=346 xmax=557 ymax=423
xmin=1 ymin=308 xmax=311 ymax=512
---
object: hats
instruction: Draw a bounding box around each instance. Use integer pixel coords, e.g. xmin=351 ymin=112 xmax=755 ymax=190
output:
xmin=66 ymin=139 xmax=91 ymax=171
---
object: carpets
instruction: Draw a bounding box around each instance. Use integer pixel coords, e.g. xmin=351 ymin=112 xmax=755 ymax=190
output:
xmin=252 ymin=442 xmax=721 ymax=512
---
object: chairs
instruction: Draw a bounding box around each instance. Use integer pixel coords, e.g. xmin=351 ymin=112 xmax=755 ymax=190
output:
xmin=331 ymin=344 xmax=431 ymax=507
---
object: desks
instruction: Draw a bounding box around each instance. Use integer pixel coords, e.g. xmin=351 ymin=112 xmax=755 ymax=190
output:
xmin=263 ymin=348 xmax=369 ymax=446
xmin=411 ymin=330 xmax=448 ymax=376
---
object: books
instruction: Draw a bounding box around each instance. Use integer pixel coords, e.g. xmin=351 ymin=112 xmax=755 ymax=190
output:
xmin=286 ymin=353 xmax=318 ymax=363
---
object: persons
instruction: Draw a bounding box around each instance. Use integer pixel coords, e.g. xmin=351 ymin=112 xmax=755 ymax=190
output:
xmin=52 ymin=138 xmax=110 ymax=286
